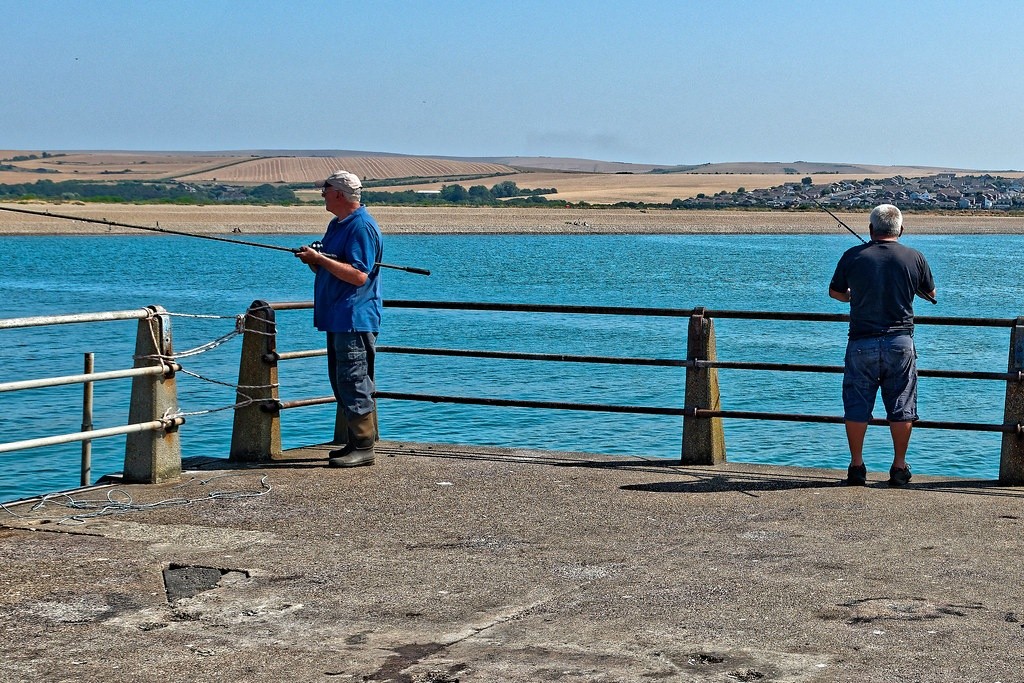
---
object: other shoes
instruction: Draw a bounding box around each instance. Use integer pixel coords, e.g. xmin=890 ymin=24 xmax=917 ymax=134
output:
xmin=329 ymin=440 xmax=355 ymax=458
xmin=329 ymin=445 xmax=376 ymax=467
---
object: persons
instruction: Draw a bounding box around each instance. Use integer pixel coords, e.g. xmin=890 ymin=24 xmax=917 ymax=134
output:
xmin=295 ymin=170 xmax=383 ymax=467
xmin=829 ymin=204 xmax=936 ymax=485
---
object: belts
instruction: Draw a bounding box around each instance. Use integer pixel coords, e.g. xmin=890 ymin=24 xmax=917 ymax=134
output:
xmin=849 ymin=331 xmax=912 ymax=337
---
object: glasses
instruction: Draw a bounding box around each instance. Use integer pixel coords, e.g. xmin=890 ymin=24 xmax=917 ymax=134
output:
xmin=322 ymin=187 xmax=339 ymax=194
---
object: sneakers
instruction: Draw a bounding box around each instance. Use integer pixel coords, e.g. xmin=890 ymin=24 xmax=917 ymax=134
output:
xmin=848 ymin=463 xmax=866 ymax=483
xmin=889 ymin=462 xmax=912 ymax=486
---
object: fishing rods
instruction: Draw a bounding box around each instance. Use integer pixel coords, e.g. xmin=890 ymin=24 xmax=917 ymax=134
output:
xmin=801 ymin=192 xmax=939 ymax=306
xmin=0 ymin=205 xmax=432 ymax=277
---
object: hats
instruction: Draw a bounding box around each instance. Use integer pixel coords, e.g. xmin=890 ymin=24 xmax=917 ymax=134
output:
xmin=314 ymin=170 xmax=362 ymax=195
